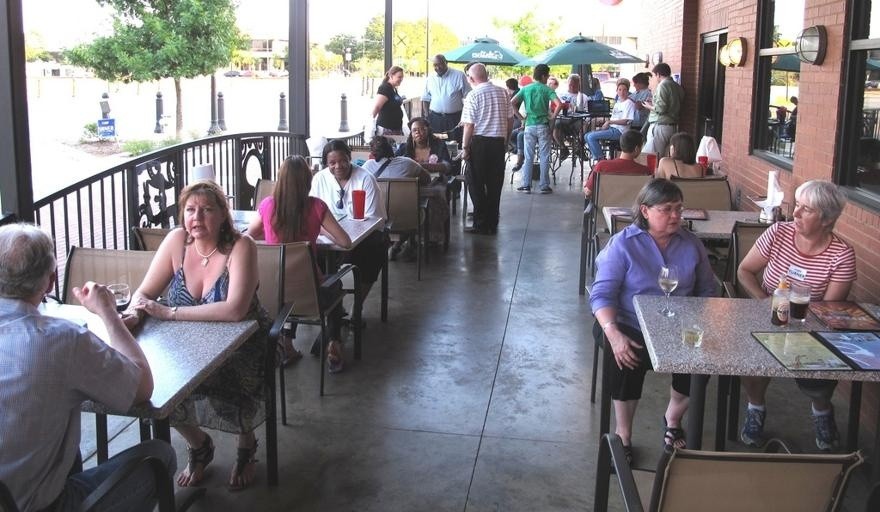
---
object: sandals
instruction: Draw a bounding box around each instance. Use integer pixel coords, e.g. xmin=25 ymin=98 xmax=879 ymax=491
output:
xmin=177 ymin=432 xmax=215 ymax=488
xmin=227 ymin=438 xmax=259 ymax=491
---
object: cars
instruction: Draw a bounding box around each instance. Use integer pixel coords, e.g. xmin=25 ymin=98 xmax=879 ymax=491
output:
xmin=225 ymin=71 xmax=240 ymax=77
xmin=239 ymin=72 xmax=254 ymax=78
xmin=269 ymin=70 xmax=290 ymax=78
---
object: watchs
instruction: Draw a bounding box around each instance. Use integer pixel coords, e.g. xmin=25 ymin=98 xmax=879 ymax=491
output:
xmin=171 ymin=306 xmax=177 ymax=319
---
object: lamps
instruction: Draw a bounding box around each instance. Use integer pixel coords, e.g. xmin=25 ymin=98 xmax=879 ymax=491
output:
xmin=719 ymin=45 xmax=733 ymax=67
xmin=797 ymin=25 xmax=828 ymax=67
xmin=730 ymin=35 xmax=747 ymax=70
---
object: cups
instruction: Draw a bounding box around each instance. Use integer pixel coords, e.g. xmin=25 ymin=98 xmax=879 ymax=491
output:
xmin=698 ymin=156 xmax=709 ymax=173
xmin=647 ymin=155 xmax=656 ymax=174
xmin=788 ymin=281 xmax=811 ymax=322
xmin=108 ymin=283 xmax=132 ymax=311
xmin=446 ymin=142 xmax=459 ymax=157
xmin=680 ymin=319 xmax=705 ymax=346
xmin=353 ymin=190 xmax=365 ymax=219
xmin=712 ymin=162 xmax=720 ymax=175
xmin=563 ymin=101 xmax=568 ymax=115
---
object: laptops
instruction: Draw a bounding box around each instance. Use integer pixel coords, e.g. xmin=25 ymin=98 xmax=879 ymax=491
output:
xmin=588 ymin=100 xmax=610 ymax=112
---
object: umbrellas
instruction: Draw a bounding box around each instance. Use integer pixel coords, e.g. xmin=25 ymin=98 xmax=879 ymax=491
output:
xmin=515 ymin=34 xmax=649 ymax=159
xmin=442 ymin=36 xmax=530 ymax=78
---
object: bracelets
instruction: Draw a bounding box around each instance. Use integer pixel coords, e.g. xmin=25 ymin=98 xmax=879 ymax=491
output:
xmin=603 ymin=321 xmax=616 ymax=330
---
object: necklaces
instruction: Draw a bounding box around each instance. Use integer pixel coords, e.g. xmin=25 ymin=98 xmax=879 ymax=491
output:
xmin=193 ymin=235 xmax=219 ymax=266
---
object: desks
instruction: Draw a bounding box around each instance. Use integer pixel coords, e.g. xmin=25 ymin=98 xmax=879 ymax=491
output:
xmin=4 ymin=297 xmax=267 ymax=510
xmin=632 ymin=285 xmax=879 ymax=457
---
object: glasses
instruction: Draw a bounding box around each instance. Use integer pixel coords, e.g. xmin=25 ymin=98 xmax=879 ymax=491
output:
xmin=336 ymin=190 xmax=344 ymax=209
xmin=653 ymin=205 xmax=684 ymax=214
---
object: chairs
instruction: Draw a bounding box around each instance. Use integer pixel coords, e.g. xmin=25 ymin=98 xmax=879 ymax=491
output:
xmin=53 ymin=239 xmax=298 ymax=487
xmin=857 ymin=138 xmax=880 ymax=189
xmin=767 ymin=107 xmax=795 ymax=158
xmin=0 ymin=128 xmax=466 ymax=512
xmin=123 ymin=128 xmax=465 ymax=400
xmin=592 ymin=427 xmax=866 ymax=509
xmin=584 ymin=166 xmax=880 ymax=396
xmin=502 ymin=93 xmax=620 ymax=191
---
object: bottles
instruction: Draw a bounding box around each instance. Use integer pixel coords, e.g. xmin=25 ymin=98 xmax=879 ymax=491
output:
xmin=759 ymin=210 xmax=774 ymax=224
xmin=770 ymin=276 xmax=789 ymax=326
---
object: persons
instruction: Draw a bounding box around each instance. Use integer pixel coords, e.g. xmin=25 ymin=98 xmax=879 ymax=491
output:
xmin=307 ymin=140 xmax=385 ymax=328
xmin=244 ymin=155 xmax=345 ymax=375
xmin=1 ymin=222 xmax=177 ymax=511
xmin=788 ymin=96 xmax=797 ymax=143
xmin=360 ymin=55 xmax=474 ymax=258
xmin=461 ymin=62 xmax=563 ymax=234
xmin=736 ymin=179 xmax=857 ymax=454
xmin=553 ymin=62 xmax=706 ymax=199
xmin=116 ymin=181 xmax=286 ymax=492
xmin=591 ymin=178 xmax=717 ymax=469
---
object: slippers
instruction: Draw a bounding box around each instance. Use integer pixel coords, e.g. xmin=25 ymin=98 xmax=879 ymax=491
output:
xmin=610 ymin=445 xmax=632 ymax=473
xmin=328 ymin=353 xmax=344 ymax=373
xmin=282 ymin=345 xmax=301 ymax=366
xmin=663 ymin=415 xmax=688 ymax=450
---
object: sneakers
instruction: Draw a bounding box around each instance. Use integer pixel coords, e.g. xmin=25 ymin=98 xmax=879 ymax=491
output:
xmin=741 ymin=406 xmax=766 ymax=449
xmin=517 ymin=186 xmax=531 ymax=193
xmin=811 ymin=410 xmax=842 ymax=451
xmin=540 ymin=187 xmax=553 ymax=194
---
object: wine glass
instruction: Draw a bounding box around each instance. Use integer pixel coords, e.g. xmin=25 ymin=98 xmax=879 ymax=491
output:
xmin=657 ymin=263 xmax=679 ymax=317
